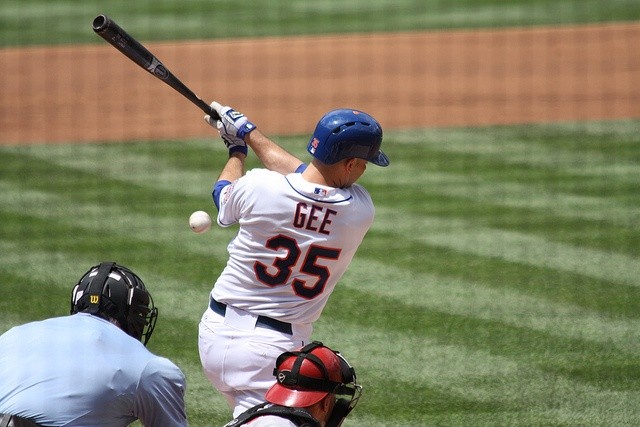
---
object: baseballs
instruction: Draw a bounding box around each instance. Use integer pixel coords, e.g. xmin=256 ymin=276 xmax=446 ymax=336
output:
xmin=188 ymin=210 xmax=212 ymax=234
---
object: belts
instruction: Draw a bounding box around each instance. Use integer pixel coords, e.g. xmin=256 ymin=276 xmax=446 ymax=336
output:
xmin=1 ymin=412 xmax=44 ymax=426
xmin=210 ymin=294 xmax=292 ymax=333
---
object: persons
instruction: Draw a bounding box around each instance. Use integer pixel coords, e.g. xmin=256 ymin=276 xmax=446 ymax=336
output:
xmin=0 ymin=262 xmax=187 ymax=427
xmin=198 ymin=101 xmax=389 ymax=419
xmin=223 ymin=341 xmax=363 ymax=427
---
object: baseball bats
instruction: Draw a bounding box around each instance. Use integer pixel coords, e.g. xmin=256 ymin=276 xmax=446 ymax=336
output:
xmin=90 ymin=15 xmax=219 ymax=120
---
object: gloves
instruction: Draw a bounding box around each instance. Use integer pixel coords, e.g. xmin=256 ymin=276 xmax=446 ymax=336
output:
xmin=222 ymin=133 xmax=248 ymax=156
xmin=203 ymin=101 xmax=257 ymax=138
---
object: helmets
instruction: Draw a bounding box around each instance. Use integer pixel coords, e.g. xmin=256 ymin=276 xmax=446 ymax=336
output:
xmin=265 ymin=341 xmax=363 ymax=427
xmin=72 ymin=263 xmax=157 ymax=347
xmin=308 ymin=107 xmax=391 ymax=166
